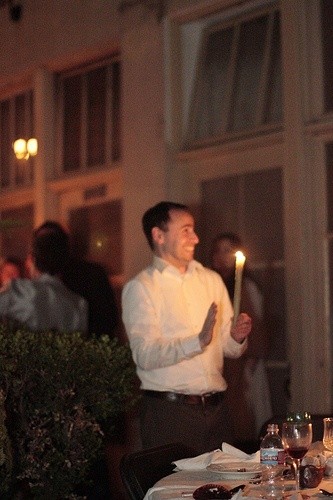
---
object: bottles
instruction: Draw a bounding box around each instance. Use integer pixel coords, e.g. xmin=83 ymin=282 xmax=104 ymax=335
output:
xmin=259 ymin=424 xmax=286 ymax=500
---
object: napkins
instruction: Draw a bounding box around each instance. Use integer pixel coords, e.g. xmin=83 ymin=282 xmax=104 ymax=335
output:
xmin=172 ymin=443 xmax=261 ymax=470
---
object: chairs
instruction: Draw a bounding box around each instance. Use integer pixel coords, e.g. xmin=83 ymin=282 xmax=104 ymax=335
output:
xmin=120 ymin=438 xmax=195 ymax=500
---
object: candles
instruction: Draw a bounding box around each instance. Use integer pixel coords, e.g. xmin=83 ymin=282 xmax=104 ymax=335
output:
xmin=233 ymin=255 xmax=248 ymax=327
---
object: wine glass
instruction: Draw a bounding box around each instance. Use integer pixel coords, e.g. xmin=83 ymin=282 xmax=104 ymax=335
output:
xmin=281 ymin=421 xmax=312 ymax=500
xmin=287 ymin=411 xmax=311 ymax=467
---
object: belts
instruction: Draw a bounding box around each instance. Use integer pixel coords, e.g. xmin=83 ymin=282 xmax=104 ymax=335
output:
xmin=143 ymin=388 xmax=220 ymax=405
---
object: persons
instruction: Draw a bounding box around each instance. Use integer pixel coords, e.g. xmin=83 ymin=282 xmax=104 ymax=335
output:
xmin=122 ymin=202 xmax=251 ymax=492
xmin=209 ymin=232 xmax=291 ymax=455
xmin=0 ymin=222 xmax=117 ymax=339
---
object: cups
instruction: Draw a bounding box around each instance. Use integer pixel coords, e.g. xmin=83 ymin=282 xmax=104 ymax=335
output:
xmin=298 ymin=456 xmax=325 ymax=488
xmin=323 ymin=417 xmax=333 ymax=452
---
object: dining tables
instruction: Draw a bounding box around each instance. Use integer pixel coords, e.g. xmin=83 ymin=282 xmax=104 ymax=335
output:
xmin=142 ymin=461 xmax=333 ymax=500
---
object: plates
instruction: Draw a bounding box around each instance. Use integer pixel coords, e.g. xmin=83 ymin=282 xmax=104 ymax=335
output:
xmin=206 ymin=462 xmax=272 ymax=479
